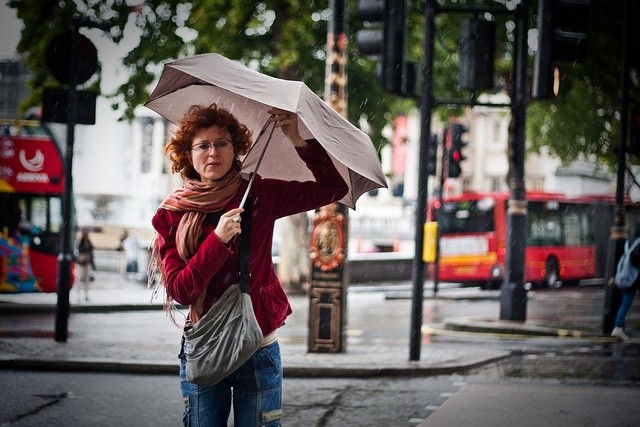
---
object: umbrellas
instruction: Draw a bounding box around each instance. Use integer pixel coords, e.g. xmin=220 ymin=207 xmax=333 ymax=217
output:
xmin=142 ymin=53 xmax=389 ymax=215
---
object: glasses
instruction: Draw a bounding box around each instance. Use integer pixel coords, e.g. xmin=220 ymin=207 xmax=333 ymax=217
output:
xmin=188 ymin=141 xmax=234 ymax=150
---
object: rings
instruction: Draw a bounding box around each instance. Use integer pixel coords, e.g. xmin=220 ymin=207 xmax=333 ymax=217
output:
xmin=230 ymin=214 xmax=241 ymax=222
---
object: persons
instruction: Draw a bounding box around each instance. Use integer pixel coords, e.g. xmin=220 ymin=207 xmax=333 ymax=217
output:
xmin=609 ymin=211 xmax=640 ymax=343
xmin=145 ymin=103 xmax=349 ymax=427
xmin=77 ymin=227 xmax=97 ymax=302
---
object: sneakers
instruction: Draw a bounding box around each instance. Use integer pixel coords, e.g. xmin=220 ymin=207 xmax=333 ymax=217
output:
xmin=612 ymin=327 xmax=629 ymax=341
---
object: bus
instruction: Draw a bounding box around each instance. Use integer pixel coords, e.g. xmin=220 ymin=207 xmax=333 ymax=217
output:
xmin=426 ymin=192 xmax=635 ymax=289
xmin=0 ymin=119 xmax=75 ymax=295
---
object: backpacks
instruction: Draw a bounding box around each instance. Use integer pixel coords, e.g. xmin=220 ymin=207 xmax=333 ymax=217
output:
xmin=615 ymin=237 xmax=640 ymax=289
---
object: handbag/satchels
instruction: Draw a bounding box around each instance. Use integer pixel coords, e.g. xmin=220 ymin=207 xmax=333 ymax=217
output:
xmin=183 ymin=283 xmax=264 ymax=387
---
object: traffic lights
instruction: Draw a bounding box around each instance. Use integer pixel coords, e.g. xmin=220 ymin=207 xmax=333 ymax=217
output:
xmin=356 ymin=0 xmax=406 ymax=96
xmin=444 ymin=124 xmax=468 ymax=176
xmin=428 ymin=133 xmax=437 ymax=175
xmin=532 ymin=0 xmax=589 ymax=99
